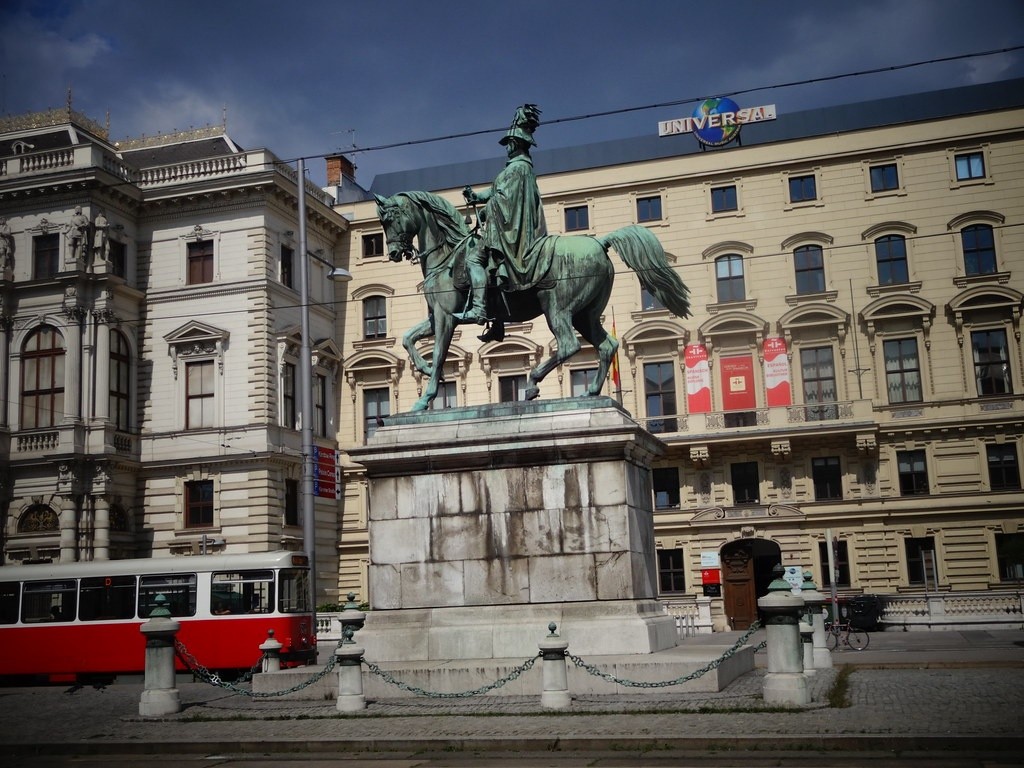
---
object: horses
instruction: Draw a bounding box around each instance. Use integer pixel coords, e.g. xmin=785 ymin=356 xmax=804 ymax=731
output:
xmin=371 ymin=189 xmax=694 ymax=411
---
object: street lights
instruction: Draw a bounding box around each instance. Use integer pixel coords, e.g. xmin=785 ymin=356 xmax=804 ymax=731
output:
xmin=296 ymin=157 xmax=354 ymax=610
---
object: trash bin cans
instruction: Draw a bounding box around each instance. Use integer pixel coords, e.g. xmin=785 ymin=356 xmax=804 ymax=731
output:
xmin=805 ymin=593 xmax=887 ymax=632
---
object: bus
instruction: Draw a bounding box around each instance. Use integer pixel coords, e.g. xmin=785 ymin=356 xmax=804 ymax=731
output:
xmin=0 ymin=549 xmax=317 ymax=689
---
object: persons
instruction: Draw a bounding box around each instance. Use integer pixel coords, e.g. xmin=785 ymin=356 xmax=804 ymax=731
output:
xmin=450 ymin=104 xmax=550 ymax=345
xmin=61 ymin=206 xmax=91 ymax=261
xmin=92 ymin=210 xmax=113 ymax=263
xmin=0 ymin=216 xmax=14 ymax=270
xmin=51 ymin=605 xmax=64 ymax=621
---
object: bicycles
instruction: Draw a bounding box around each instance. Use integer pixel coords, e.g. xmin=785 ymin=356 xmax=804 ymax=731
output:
xmin=823 ymin=618 xmax=871 ymax=653
